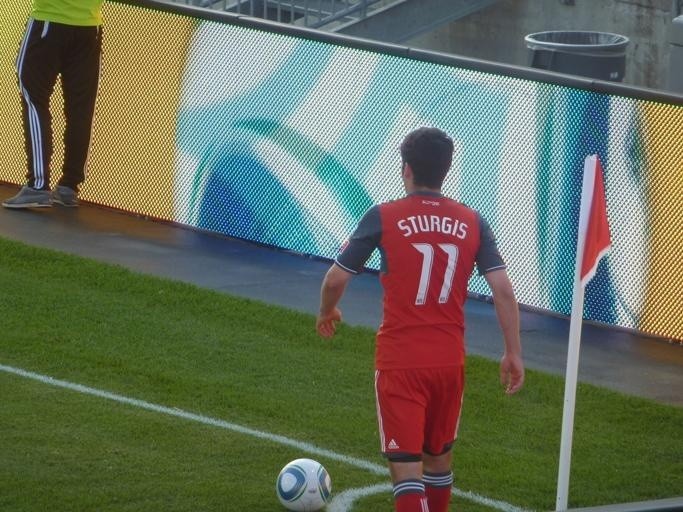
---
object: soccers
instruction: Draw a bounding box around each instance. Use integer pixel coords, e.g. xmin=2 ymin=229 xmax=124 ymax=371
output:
xmin=275 ymin=458 xmax=331 ymax=511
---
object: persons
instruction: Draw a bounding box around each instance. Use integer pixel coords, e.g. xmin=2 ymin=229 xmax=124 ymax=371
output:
xmin=0 ymin=0 xmax=104 ymax=209
xmin=314 ymin=126 xmax=526 ymax=512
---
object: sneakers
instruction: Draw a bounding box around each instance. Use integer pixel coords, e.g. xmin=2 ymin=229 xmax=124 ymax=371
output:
xmin=46 ymin=184 xmax=80 ymax=209
xmin=2 ymin=184 xmax=54 ymax=209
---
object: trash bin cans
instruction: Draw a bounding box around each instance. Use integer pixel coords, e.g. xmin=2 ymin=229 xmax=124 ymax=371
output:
xmin=524 ymin=31 xmax=630 ymax=82
